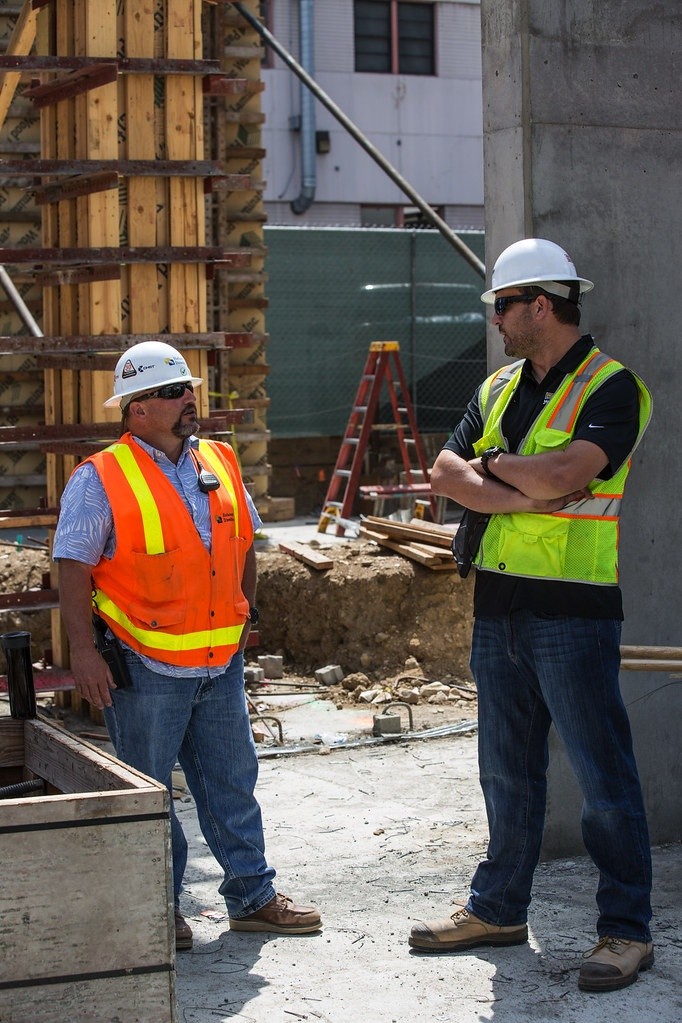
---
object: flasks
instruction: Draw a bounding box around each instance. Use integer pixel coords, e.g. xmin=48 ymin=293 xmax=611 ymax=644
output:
xmin=0 ymin=631 xmax=37 ymax=719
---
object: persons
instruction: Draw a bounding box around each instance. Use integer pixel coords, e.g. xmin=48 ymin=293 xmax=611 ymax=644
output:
xmin=52 ymin=341 xmax=321 ymax=948
xmin=409 ymin=237 xmax=657 ymax=993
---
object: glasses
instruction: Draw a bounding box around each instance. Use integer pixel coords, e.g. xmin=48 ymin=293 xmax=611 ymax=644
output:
xmin=124 ymin=381 xmax=194 ymax=412
xmin=494 ymin=294 xmax=556 ymax=315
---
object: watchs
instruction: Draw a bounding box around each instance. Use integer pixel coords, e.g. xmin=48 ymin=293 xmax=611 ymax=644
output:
xmin=478 ymin=446 xmax=508 ymax=480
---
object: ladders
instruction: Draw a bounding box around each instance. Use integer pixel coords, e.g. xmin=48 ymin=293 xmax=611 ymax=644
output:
xmin=317 ymin=340 xmax=438 ymax=538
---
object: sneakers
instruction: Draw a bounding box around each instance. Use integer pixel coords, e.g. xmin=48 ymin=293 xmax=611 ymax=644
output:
xmin=229 ymin=893 xmax=322 ymax=934
xmin=174 ymin=907 xmax=194 ymax=948
xmin=577 ymin=935 xmax=654 ymax=990
xmin=408 ymin=908 xmax=528 ymax=952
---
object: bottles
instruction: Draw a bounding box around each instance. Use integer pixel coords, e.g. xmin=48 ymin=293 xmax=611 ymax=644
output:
xmin=16 ymin=534 xmax=23 ymax=551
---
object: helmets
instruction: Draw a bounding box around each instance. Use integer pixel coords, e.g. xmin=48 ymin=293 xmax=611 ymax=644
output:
xmin=480 ymin=239 xmax=595 ymax=305
xmin=102 ymin=341 xmax=205 ymax=409
